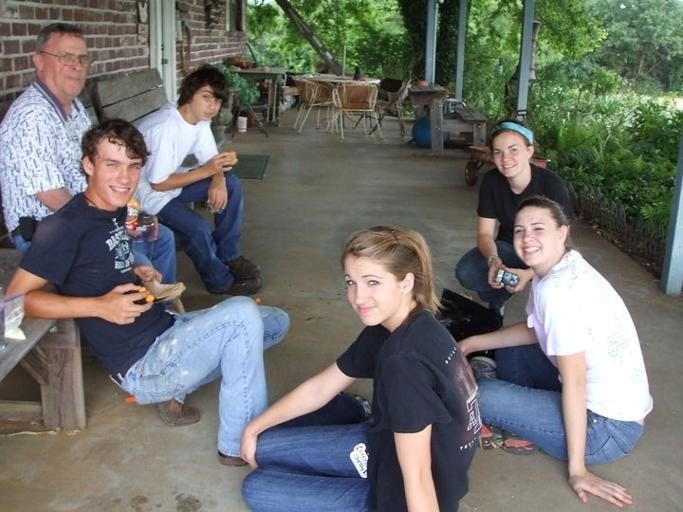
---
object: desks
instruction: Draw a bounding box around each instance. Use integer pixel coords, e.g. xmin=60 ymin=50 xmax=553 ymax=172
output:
xmin=228 ymin=65 xmax=287 ymax=126
xmin=406 ymin=80 xmax=447 ymax=154
xmin=307 ymin=73 xmax=380 ymax=136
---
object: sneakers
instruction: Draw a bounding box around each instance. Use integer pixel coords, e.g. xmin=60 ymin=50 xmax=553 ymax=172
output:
xmin=170 ymin=300 xmax=186 ymax=314
xmin=474 ymin=426 xmax=542 ymax=456
xmin=140 ymin=280 xmax=186 ymax=300
xmin=226 ymin=256 xmax=259 ymax=277
xmin=340 ymin=388 xmax=373 ymax=419
xmin=466 ymin=357 xmax=497 ymax=378
xmin=216 ymin=453 xmax=246 ymax=468
xmin=158 ymin=401 xmax=199 ymax=428
xmin=210 ymin=276 xmax=262 ymax=294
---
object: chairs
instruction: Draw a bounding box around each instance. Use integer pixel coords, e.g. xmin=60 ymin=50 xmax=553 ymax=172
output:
xmin=93 ymin=67 xmax=225 ymax=213
xmin=0 ymin=246 xmax=88 ymax=437
xmin=327 ymin=81 xmax=384 ymax=141
xmin=368 ymin=77 xmax=412 ymax=140
xmin=291 ymin=77 xmax=327 ymax=133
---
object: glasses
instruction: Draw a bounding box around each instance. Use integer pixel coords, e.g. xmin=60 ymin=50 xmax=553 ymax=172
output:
xmin=368 ymin=224 xmax=403 ymax=247
xmin=37 ymin=52 xmax=92 ymax=66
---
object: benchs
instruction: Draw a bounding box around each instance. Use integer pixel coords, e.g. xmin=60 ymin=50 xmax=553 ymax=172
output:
xmin=448 ymin=103 xmax=488 ymax=151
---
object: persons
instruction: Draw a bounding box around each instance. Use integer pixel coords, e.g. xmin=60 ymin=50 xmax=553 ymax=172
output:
xmin=240 ymin=224 xmax=484 ymax=512
xmin=130 ymin=62 xmax=263 ymax=295
xmin=0 ymin=116 xmax=290 ymax=468
xmin=0 ymin=24 xmax=185 ymax=316
xmin=454 ymin=118 xmax=576 ymax=320
xmin=453 ymin=195 xmax=655 ymax=509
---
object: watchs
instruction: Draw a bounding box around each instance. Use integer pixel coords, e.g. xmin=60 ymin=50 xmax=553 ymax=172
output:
xmin=486 ymin=254 xmax=502 ymax=268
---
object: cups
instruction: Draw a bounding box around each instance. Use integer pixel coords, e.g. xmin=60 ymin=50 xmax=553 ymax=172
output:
xmin=140 ymin=216 xmax=159 ymax=243
